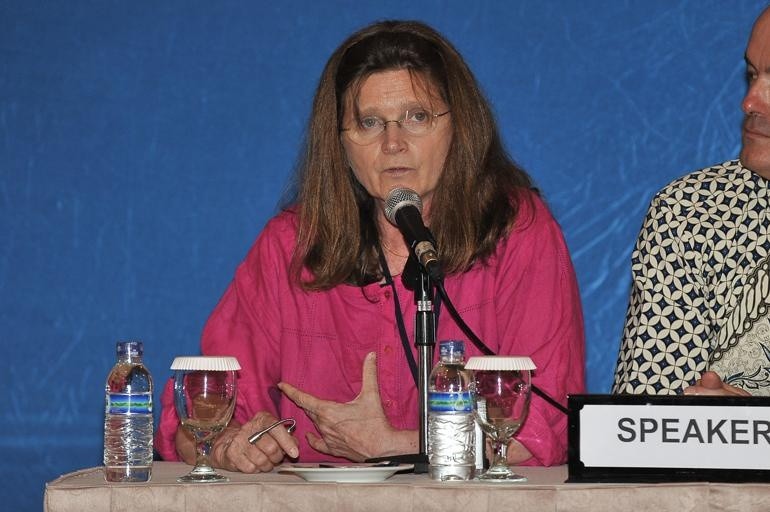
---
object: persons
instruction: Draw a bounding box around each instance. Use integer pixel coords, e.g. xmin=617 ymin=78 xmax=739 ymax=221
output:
xmin=611 ymin=5 xmax=770 ymax=397
xmin=156 ymin=19 xmax=587 ymax=475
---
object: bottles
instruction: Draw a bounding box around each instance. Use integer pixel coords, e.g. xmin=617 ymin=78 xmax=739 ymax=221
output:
xmin=103 ymin=339 xmax=155 ymax=482
xmin=428 ymin=339 xmax=477 ymax=480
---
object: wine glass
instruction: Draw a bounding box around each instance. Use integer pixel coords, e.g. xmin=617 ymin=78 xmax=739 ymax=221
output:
xmin=174 ymin=370 xmax=237 ymax=482
xmin=467 ymin=350 xmax=532 ymax=482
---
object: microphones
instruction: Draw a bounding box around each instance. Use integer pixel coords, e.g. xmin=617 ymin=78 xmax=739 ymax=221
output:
xmin=381 ymin=184 xmax=445 ymax=285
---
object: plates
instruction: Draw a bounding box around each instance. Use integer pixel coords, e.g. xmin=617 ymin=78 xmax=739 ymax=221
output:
xmin=280 ymin=462 xmax=415 ymax=483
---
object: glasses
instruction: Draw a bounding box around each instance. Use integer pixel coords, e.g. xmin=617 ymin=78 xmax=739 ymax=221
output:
xmin=339 ymin=105 xmax=454 ymax=147
